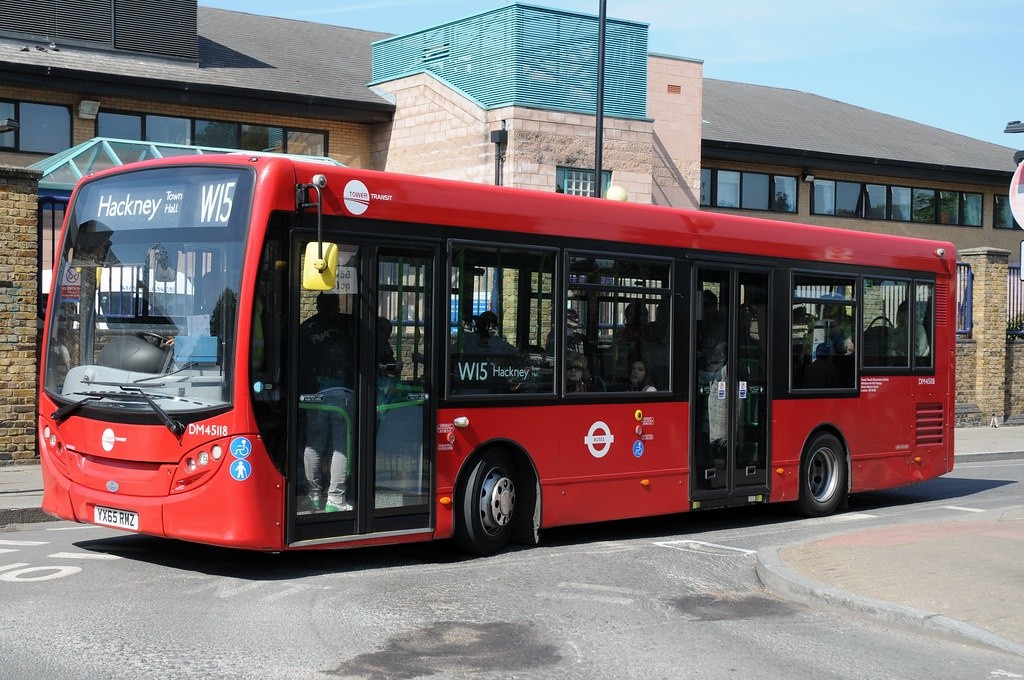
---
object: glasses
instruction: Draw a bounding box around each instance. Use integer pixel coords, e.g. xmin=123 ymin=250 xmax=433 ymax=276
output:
xmin=567 ymin=366 xmax=583 ymax=371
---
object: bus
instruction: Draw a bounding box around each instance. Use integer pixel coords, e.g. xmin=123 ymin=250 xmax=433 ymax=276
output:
xmin=37 ymin=152 xmax=959 ymax=557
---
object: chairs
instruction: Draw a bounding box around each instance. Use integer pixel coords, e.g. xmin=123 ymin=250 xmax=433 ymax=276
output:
xmin=864 ymin=317 xmax=894 ymax=366
xmin=585 ymin=375 xmax=607 ymax=392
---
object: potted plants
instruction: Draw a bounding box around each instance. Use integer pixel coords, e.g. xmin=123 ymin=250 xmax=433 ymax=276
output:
xmin=912 ymin=191 xmax=968 ymax=224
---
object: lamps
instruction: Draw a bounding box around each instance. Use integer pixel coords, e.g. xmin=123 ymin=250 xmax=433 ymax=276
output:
xmin=0 ymin=118 xmax=20 ymax=133
xmin=77 ymin=100 xmax=100 ymax=120
xmin=802 ymin=169 xmax=814 ymax=182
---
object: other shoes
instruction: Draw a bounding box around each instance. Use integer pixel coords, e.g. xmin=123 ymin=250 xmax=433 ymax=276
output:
xmin=299 ymin=493 xmax=321 ymax=510
xmin=325 ymin=499 xmax=353 ymax=512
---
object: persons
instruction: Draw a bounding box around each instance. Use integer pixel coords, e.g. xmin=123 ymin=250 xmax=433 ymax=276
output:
xmin=449 ymin=275 xmax=690 ymax=392
xmin=697 ymin=289 xmax=766 ymax=469
xmin=792 ymin=313 xmax=855 ymax=388
xmin=300 ymin=293 xmax=361 ymax=513
xmin=376 ymin=316 xmax=404 ymax=374
xmin=165 ymin=271 xmax=221 ymax=348
xmin=886 ymin=301 xmax=930 ymax=356
